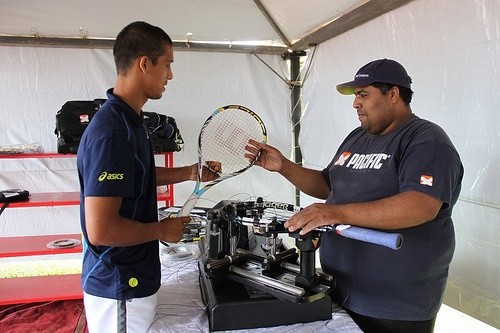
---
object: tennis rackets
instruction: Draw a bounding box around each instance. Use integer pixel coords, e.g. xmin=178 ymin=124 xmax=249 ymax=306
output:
xmin=220 ymin=201 xmax=403 ymax=251
xmin=160 ymin=105 xmax=268 ymax=247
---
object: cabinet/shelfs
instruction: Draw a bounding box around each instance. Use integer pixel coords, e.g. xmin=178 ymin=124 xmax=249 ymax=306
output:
xmin=0 ymin=149 xmax=176 ymax=307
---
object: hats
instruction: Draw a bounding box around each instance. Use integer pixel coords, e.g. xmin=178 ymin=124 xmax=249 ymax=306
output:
xmin=336 ymin=58 xmax=412 ymax=96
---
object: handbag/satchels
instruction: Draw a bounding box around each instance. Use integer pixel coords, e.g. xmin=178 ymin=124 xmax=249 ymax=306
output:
xmin=53 ymin=98 xmax=108 ymax=155
xmin=141 ymin=111 xmax=185 ymax=155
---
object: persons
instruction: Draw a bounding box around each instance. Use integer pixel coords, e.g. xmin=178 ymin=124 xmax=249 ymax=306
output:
xmin=76 ymin=21 xmax=221 ymax=333
xmin=246 ymin=58 xmax=465 ymax=333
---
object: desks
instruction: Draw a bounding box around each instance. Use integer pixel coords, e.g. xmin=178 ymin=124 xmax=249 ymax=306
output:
xmin=147 ymin=225 xmax=363 ymax=333
